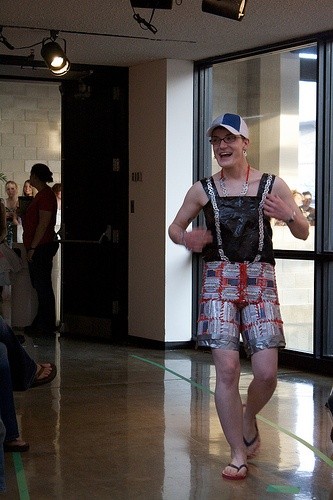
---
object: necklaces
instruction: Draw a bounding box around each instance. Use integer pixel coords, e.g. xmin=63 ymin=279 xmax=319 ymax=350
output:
xmin=220 ymin=163 xmax=250 ymax=197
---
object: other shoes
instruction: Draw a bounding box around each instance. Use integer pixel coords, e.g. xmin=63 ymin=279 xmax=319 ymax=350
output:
xmin=24 ymin=313 xmax=56 ymax=334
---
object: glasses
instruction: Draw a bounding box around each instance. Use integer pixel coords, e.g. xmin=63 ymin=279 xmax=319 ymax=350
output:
xmin=209 ymin=133 xmax=241 ymax=145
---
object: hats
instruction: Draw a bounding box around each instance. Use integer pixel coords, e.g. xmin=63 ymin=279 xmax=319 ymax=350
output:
xmin=206 ymin=113 xmax=250 ymax=140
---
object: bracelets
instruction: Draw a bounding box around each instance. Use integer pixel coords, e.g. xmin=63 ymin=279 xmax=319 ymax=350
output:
xmin=287 ymin=212 xmax=295 ymax=224
xmin=30 ymin=247 xmax=36 ymax=250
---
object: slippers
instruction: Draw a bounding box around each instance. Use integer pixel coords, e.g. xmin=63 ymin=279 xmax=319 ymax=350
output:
xmin=14 ymin=361 xmax=56 ymax=390
xmin=3 ymin=436 xmax=30 ymax=453
xmin=241 ymin=404 xmax=260 ymax=457
xmin=222 ymin=454 xmax=248 ymax=479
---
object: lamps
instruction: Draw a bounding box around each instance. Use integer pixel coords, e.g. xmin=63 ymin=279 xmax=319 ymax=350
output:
xmin=130 ymin=0 xmax=173 ymax=34
xmin=202 ymin=0 xmax=247 ymax=22
xmin=41 ymin=29 xmax=70 ymax=76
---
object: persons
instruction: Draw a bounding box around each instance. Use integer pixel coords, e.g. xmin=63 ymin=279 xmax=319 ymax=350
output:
xmin=168 ymin=112 xmax=309 ymax=479
xmin=0 ymin=202 xmax=22 ymax=300
xmin=14 ymin=180 xmax=38 ymax=225
xmin=293 ymin=190 xmax=315 ymax=225
xmin=0 ymin=315 xmax=57 ymax=450
xmin=52 ymin=183 xmax=62 ymax=240
xmin=23 ymin=163 xmax=58 ymax=335
xmin=2 ymin=181 xmax=18 ymax=249
